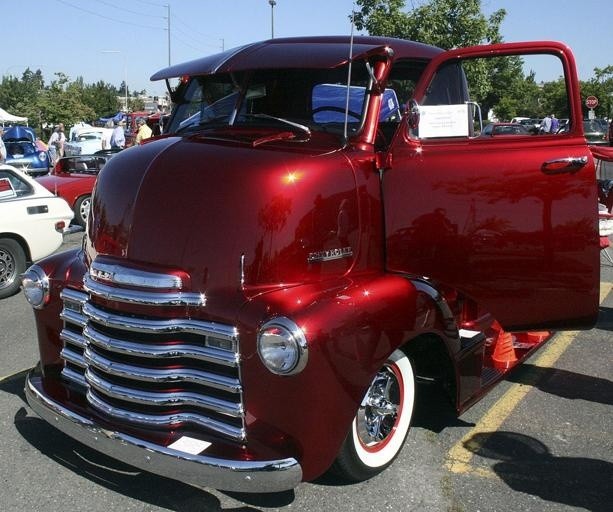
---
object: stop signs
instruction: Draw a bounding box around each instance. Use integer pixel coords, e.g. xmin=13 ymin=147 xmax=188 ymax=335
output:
xmin=584 ymin=94 xmax=598 ymax=109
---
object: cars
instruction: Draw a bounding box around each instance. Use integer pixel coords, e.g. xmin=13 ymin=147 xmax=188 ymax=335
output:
xmin=0 ymin=111 xmax=159 ymax=299
xmin=482 ymin=109 xmax=613 ymax=218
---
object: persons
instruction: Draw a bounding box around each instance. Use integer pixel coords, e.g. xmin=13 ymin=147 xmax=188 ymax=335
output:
xmin=99 ymin=119 xmax=115 ymax=151
xmin=46 ymin=125 xmax=62 ymax=165
xmin=133 ymin=118 xmax=155 ymax=145
xmin=549 ymin=113 xmax=559 ymax=133
xmin=109 ymin=116 xmax=126 ymax=152
xmin=55 ymin=124 xmax=66 ymax=158
xmin=0 ymin=126 xmax=6 ymax=165
xmin=537 ymin=114 xmax=551 ymax=134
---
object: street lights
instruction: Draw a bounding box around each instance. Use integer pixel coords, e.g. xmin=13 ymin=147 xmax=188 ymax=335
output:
xmin=100 ymin=49 xmax=127 ymax=108
xmin=162 ymin=27 xmax=171 ymax=67
xmin=268 ymin=0 xmax=276 ymax=38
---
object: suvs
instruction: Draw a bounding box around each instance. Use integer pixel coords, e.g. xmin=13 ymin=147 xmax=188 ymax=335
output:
xmin=16 ymin=25 xmax=605 ymax=501
